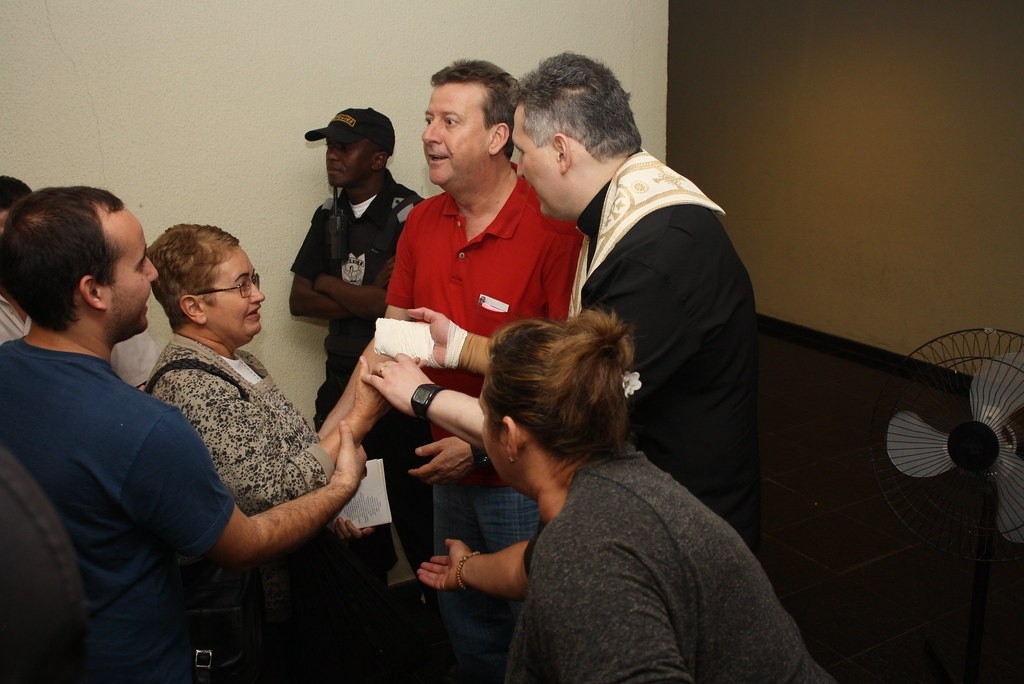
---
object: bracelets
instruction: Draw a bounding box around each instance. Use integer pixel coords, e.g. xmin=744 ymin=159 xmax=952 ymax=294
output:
xmin=456 ymin=551 xmax=480 ymax=589
xmin=471 ymin=444 xmax=493 ymax=470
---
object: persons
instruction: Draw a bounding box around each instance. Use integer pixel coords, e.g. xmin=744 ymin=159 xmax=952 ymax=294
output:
xmin=0 ymin=174 xmax=367 ymax=684
xmin=289 ymin=107 xmax=426 ymax=578
xmin=405 ymin=51 xmax=761 ymax=559
xmin=318 ymin=57 xmax=590 ymax=684
xmin=140 ymin=223 xmax=432 ymax=683
xmin=417 ymin=306 xmax=844 ymax=683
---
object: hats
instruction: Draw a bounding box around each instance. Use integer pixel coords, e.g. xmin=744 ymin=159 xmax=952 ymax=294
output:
xmin=305 ymin=108 xmax=396 ymax=156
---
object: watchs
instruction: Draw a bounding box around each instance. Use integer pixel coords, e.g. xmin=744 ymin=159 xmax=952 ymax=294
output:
xmin=410 ymin=384 xmax=443 ymax=417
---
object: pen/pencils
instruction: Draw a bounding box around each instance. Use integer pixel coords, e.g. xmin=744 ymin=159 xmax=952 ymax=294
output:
xmin=478 ymin=296 xmax=486 ymax=305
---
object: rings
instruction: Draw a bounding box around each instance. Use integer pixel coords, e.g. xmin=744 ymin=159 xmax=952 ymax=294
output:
xmin=380 ymin=366 xmax=387 ymax=372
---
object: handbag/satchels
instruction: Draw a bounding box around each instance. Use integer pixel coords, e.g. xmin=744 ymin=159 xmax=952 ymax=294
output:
xmin=185 ymin=564 xmax=262 ymax=684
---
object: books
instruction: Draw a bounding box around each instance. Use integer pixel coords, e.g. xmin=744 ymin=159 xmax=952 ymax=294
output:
xmin=338 ymin=459 xmax=392 ymax=529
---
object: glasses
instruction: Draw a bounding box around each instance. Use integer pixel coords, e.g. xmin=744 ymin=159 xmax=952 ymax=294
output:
xmin=190 ymin=273 xmax=261 ymax=298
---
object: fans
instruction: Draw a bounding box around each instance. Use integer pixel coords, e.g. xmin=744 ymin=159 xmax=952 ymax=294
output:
xmin=867 ymin=327 xmax=1024 ymax=684
